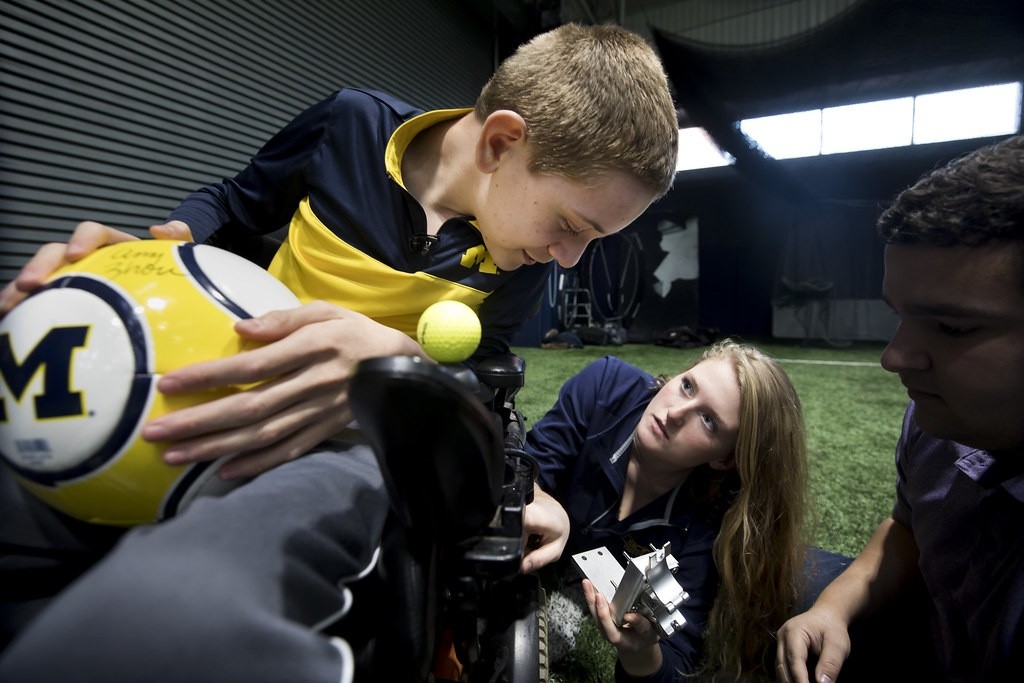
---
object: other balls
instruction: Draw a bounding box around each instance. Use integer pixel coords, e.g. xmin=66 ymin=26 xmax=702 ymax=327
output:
xmin=415 ymin=300 xmax=483 ymax=365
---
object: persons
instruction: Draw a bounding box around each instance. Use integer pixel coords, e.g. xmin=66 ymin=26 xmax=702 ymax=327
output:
xmin=521 ymin=342 xmax=800 ymax=683
xmin=780 ymin=138 xmax=1024 ymax=683
xmin=0 ymin=23 xmax=678 ymax=683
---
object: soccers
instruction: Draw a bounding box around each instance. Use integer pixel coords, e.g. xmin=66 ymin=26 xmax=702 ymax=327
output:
xmin=1 ymin=236 xmax=306 ymax=529
